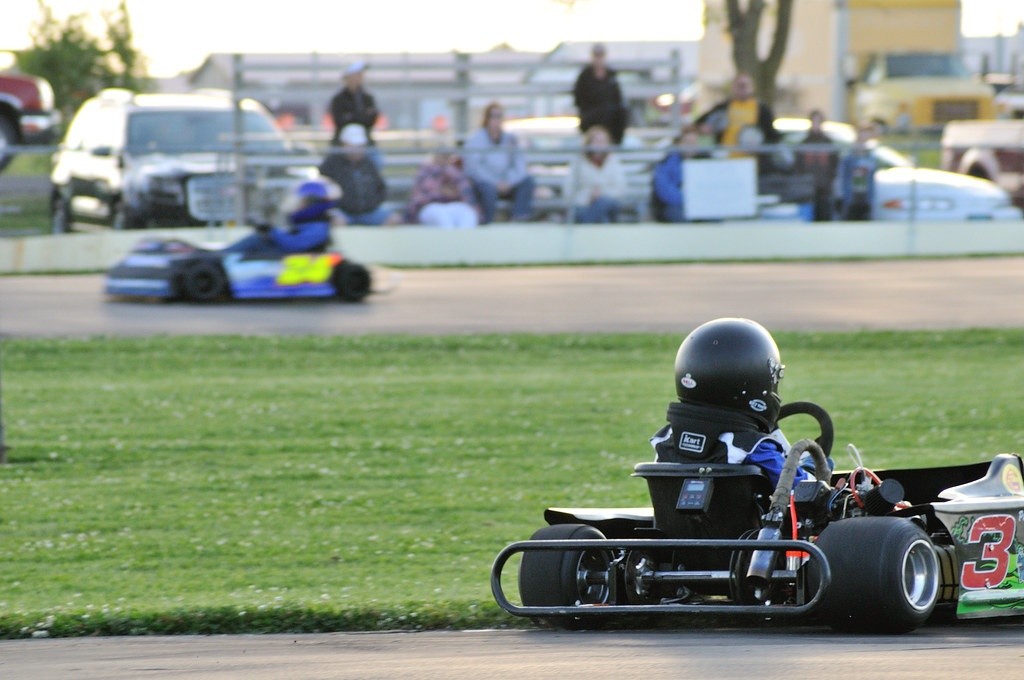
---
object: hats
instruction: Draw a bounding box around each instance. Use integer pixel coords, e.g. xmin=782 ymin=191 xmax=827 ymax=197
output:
xmin=339 ymin=123 xmax=367 ymax=146
xmin=340 ymin=58 xmax=370 ymax=80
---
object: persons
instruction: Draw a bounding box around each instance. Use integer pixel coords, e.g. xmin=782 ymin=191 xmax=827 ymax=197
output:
xmin=322 ymin=122 xmax=389 ymax=214
xmin=560 ymin=124 xmax=625 ymax=223
xmin=402 ymin=140 xmax=485 ymax=224
xmin=225 ymin=182 xmax=334 ymax=259
xmin=573 ymin=47 xmax=627 ymax=144
xmin=837 ymin=125 xmax=883 ymax=220
xmin=650 ymin=318 xmax=835 ymax=490
xmin=722 ymin=73 xmax=778 ymax=169
xmin=793 ymin=112 xmax=840 ymax=219
xmin=655 ymin=105 xmax=726 ymax=223
xmin=329 ymin=63 xmax=379 ymax=137
xmin=461 ymin=104 xmax=535 ymax=223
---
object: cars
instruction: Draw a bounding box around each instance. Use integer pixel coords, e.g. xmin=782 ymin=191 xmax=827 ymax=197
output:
xmin=774 ymin=117 xmax=1023 ymax=223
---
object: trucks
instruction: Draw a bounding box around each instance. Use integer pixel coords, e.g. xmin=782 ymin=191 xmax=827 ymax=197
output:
xmin=691 ymin=0 xmax=996 ymax=159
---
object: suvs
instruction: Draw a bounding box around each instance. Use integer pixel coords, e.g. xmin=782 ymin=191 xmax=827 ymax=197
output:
xmin=51 ymin=85 xmax=318 ymax=234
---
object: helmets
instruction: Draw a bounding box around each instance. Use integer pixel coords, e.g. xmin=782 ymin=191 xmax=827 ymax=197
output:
xmin=284 ymin=181 xmax=344 ymax=225
xmin=674 ymin=317 xmax=785 ymax=433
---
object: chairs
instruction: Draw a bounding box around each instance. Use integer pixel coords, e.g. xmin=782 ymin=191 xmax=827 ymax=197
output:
xmin=630 ymin=460 xmax=772 ymax=570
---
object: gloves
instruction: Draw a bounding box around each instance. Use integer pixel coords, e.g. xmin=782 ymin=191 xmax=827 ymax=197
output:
xmin=801 ymin=455 xmax=835 ymax=478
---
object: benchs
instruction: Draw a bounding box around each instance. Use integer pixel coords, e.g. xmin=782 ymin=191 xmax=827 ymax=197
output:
xmin=237 ymin=153 xmax=667 ymax=223
